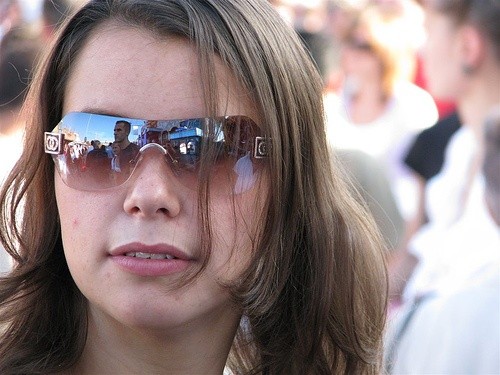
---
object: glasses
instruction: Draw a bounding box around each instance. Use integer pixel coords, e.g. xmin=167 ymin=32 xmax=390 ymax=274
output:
xmin=39 ymin=108 xmax=272 ymax=196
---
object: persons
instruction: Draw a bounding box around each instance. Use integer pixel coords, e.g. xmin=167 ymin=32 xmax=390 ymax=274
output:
xmin=173 ymin=145 xmax=182 ymax=158
xmin=271 ymin=0 xmax=499 ymax=374
xmin=58 ymin=136 xmax=113 ymax=175
xmin=0 ymin=0 xmax=90 ymax=274
xmin=0 ymin=0 xmax=389 ymax=375
xmin=111 ymin=141 xmax=122 ymax=173
xmin=113 ymin=120 xmax=140 ymax=180
xmin=166 ymin=143 xmax=176 ymax=159
xmin=86 ymin=139 xmax=109 ymax=183
